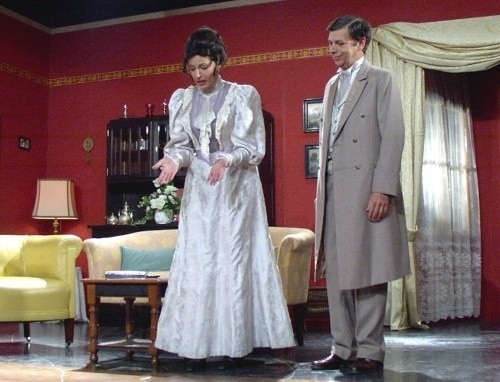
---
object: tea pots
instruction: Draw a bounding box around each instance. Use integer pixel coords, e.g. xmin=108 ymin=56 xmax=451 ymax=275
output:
xmin=105 ymin=212 xmax=119 ymax=225
xmin=118 ymin=201 xmax=134 ymax=225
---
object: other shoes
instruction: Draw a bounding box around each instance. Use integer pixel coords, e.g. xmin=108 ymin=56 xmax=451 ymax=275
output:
xmin=340 ymin=358 xmax=383 ymax=375
xmin=180 ymin=359 xmax=205 ymax=371
xmin=311 ymin=354 xmax=354 ymax=369
xmin=216 ymin=358 xmax=242 ymax=370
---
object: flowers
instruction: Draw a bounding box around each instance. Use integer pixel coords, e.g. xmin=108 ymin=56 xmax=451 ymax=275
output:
xmin=138 ymin=184 xmax=181 ymax=220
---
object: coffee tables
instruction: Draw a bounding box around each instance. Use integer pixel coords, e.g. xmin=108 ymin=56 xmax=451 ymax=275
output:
xmin=81 ymin=273 xmax=169 ymax=376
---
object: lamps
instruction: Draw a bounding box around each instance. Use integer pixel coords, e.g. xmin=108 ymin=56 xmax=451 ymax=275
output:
xmin=32 ymin=178 xmax=80 ymax=235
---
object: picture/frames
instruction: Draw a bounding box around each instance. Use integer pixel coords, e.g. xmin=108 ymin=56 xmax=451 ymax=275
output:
xmin=303 ymin=98 xmax=323 ymax=132
xmin=305 ymin=144 xmax=319 ymax=178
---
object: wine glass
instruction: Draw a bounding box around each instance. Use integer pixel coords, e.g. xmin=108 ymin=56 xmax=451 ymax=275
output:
xmin=123 ymin=104 xmax=129 ymax=118
xmin=146 ymin=104 xmax=155 ymax=116
xmin=159 ymin=100 xmax=169 ymax=114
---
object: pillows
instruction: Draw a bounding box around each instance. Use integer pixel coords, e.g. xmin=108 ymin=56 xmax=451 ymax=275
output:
xmin=120 ymin=247 xmax=175 ymax=271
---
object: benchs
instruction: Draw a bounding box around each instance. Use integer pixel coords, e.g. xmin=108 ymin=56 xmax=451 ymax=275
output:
xmin=81 ymin=226 xmax=315 ymax=353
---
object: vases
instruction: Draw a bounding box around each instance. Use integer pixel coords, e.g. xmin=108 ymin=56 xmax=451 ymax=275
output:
xmin=155 ymin=209 xmax=173 ymax=224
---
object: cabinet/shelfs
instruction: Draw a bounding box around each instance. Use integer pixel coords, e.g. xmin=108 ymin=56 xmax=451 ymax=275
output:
xmin=87 ymin=110 xmax=275 ymax=238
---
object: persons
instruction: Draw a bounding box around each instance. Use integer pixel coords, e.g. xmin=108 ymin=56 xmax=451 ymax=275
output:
xmin=152 ymin=27 xmax=297 ymax=371
xmin=310 ymin=16 xmax=412 ymax=375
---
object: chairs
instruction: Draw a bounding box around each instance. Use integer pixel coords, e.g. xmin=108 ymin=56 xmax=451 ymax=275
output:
xmin=0 ymin=234 xmax=83 ymax=346
